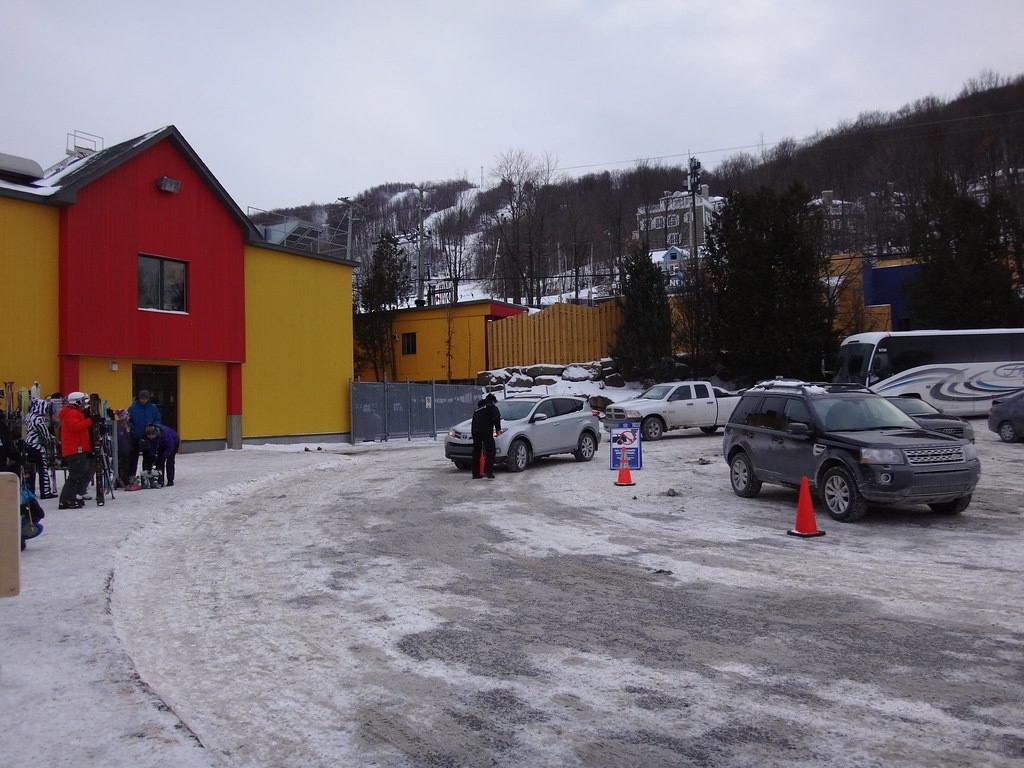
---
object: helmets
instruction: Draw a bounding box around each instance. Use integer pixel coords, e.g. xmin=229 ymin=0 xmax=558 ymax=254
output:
xmin=68 ymin=392 xmax=88 ymax=404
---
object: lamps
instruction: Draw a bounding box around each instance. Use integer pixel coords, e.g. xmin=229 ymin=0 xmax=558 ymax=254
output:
xmin=160 ymin=176 xmax=180 ymax=193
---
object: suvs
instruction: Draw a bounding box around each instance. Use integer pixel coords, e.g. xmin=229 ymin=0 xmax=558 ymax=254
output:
xmin=444 ymin=393 xmax=602 ymax=472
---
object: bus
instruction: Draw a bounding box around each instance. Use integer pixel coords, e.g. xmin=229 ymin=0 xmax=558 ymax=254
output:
xmin=829 ymin=328 xmax=1024 ymax=420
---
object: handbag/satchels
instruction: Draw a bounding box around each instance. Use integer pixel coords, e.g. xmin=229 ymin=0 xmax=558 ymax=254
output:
xmin=150 ymin=468 xmax=163 ymax=489
xmin=140 ymin=469 xmax=150 ymax=488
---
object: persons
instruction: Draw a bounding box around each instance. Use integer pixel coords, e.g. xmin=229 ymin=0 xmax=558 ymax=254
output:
xmin=0 ymin=388 xmax=180 ymax=550
xmin=469 ymin=390 xmax=502 ymax=481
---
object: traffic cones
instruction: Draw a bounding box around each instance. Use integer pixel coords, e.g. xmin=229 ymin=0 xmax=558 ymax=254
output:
xmin=612 ymin=446 xmax=637 ymax=487
xmin=478 ymin=450 xmax=486 ymax=478
xmin=786 ymin=476 xmax=827 ymax=538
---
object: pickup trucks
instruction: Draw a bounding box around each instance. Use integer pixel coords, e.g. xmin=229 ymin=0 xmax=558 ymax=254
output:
xmin=605 ymin=380 xmax=743 ymax=441
xmin=721 ymin=382 xmax=981 ymax=524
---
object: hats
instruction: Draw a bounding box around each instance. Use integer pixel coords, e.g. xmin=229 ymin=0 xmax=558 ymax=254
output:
xmin=138 ymin=390 xmax=150 ymax=399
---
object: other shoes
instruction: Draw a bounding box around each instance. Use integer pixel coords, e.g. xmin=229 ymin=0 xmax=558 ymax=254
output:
xmin=59 ymin=497 xmax=85 ymax=509
xmin=486 ymin=473 xmax=495 ymax=478
xmin=82 ymin=494 xmax=92 ymax=500
xmin=76 ymin=494 xmax=83 ymax=500
xmin=40 ymin=492 xmax=58 ymax=499
xmin=473 ymin=474 xmax=482 ymax=479
xmin=167 ymin=480 xmax=174 ymax=486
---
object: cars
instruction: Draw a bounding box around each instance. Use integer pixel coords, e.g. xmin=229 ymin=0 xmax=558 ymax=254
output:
xmin=987 ymin=389 xmax=1024 ymax=442
xmin=883 ymin=395 xmax=977 ymax=446
